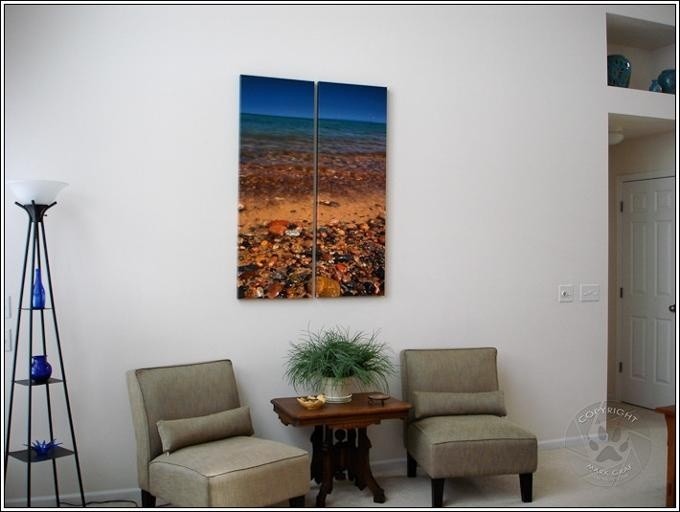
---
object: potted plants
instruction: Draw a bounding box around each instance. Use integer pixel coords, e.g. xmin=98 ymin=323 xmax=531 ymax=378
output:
xmin=281 ymin=322 xmax=399 ymax=404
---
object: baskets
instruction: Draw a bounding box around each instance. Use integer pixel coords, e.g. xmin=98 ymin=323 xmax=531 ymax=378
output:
xmin=296 ymin=393 xmax=326 ymax=410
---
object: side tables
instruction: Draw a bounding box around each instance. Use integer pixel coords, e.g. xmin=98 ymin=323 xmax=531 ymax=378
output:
xmin=271 ymin=392 xmax=414 ymax=507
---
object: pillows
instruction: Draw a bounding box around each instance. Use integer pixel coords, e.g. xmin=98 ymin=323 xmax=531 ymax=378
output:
xmin=157 ymin=406 xmax=253 ymax=451
xmin=410 ymin=390 xmax=506 ymax=418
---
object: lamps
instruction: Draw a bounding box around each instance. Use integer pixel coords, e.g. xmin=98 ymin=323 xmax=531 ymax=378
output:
xmin=5 ymin=181 xmax=88 ymax=507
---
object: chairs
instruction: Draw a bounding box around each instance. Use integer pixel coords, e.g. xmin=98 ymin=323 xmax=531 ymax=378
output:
xmin=400 ymin=347 xmax=538 ymax=507
xmin=125 ymin=359 xmax=310 ymax=508
